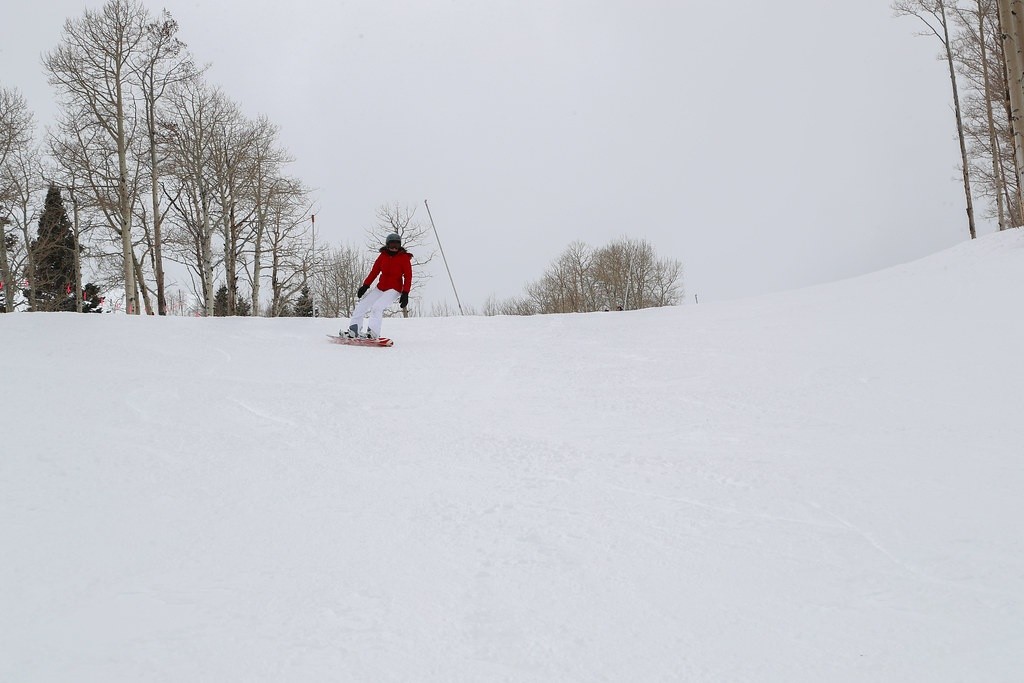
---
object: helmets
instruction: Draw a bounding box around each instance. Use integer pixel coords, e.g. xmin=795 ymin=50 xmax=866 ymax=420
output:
xmin=385 ymin=234 xmax=401 ymax=243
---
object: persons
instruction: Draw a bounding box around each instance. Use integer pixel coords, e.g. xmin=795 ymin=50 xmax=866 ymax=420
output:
xmin=340 ymin=233 xmax=413 ymax=341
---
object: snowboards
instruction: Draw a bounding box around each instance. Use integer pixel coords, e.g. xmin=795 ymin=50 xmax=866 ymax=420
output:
xmin=326 ymin=333 xmax=394 ymax=348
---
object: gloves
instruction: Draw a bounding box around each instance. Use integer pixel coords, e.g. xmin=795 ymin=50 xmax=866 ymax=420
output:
xmin=399 ymin=291 xmax=409 ymax=308
xmin=357 ymin=285 xmax=370 ymax=298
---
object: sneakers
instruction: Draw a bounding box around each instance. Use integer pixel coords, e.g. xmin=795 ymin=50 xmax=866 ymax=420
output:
xmin=365 ymin=327 xmax=377 ymax=339
xmin=339 ymin=324 xmax=360 ymax=339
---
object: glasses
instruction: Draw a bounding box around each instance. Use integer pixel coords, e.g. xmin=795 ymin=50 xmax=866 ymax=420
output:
xmin=389 ymin=242 xmax=400 ymax=249
